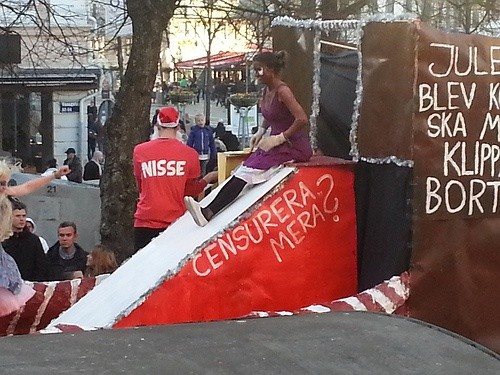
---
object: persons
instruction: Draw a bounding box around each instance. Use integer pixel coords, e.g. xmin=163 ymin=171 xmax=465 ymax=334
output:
xmin=151 ymin=109 xmax=241 ymax=202
xmin=63 ymin=148 xmax=82 ymax=183
xmin=47 ymin=222 xmax=89 ymax=280
xmin=133 ymin=107 xmax=201 ymax=252
xmin=0 ymin=161 xmax=71 ymax=199
xmin=84 ymin=245 xmax=117 ymax=276
xmin=83 ymin=150 xmax=103 ymax=181
xmin=1 ymin=202 xmax=53 ymax=282
xmin=155 ymin=77 xmax=245 ymax=107
xmin=184 ymin=51 xmax=311 ymax=227
xmin=38 ymin=147 xmax=56 ymax=173
xmin=87 ymin=101 xmax=102 ymax=157
xmin=0 ymin=198 xmax=70 ymax=317
xmin=25 ymin=218 xmax=49 ymax=254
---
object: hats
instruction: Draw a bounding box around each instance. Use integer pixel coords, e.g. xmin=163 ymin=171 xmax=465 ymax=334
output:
xmin=157 ymin=107 xmax=179 ymax=128
xmin=64 ymin=148 xmax=75 ymax=153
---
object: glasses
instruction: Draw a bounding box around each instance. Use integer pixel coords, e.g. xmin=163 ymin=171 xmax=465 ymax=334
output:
xmin=0 ymin=181 xmax=10 ymax=187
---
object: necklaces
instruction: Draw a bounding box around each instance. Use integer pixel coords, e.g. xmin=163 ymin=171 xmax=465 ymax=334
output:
xmin=267 ymin=86 xmax=275 ymax=96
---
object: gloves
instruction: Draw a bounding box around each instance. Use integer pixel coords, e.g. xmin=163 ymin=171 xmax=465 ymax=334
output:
xmin=250 ymin=127 xmax=266 ymax=151
xmin=257 ymin=132 xmax=287 ymax=152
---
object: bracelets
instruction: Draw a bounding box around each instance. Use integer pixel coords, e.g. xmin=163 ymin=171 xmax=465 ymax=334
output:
xmin=52 ymin=171 xmax=61 ymax=179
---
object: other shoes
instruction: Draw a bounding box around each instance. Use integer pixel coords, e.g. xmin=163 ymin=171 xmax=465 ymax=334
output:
xmin=188 ymin=196 xmax=208 ymax=227
xmin=184 ymin=196 xmax=200 ymax=226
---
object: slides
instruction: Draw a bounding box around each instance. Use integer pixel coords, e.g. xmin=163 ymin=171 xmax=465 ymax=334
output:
xmin=37 ymin=161 xmax=298 ymax=334
